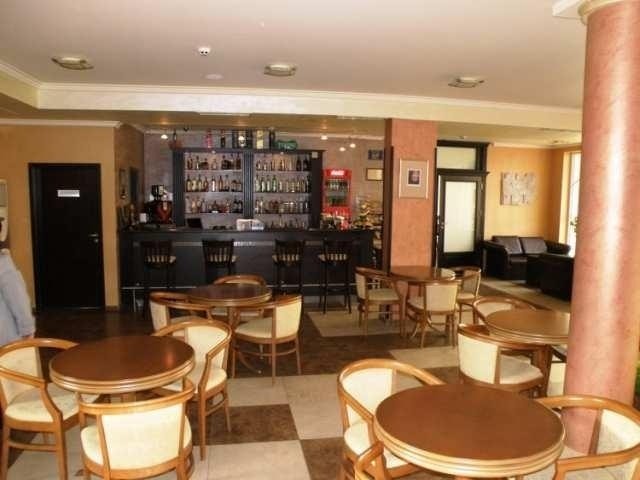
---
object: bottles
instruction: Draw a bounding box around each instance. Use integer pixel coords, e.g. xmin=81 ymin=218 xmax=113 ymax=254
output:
xmin=232 ymin=129 xmax=252 ymax=148
xmin=327 ymin=179 xmax=346 ymax=207
xmin=254 ymin=196 xmax=310 ymax=214
xmin=184 ymin=195 xmax=243 ymax=214
xmin=255 ymin=174 xmax=311 ymax=193
xmin=257 ymin=155 xmax=309 ymax=171
xmin=271 ymin=218 xmax=305 ymax=230
xmin=256 ymin=129 xmax=264 ymax=149
xmin=268 ymin=129 xmax=276 ymax=149
xmin=207 ymin=128 xmax=213 ymax=148
xmin=221 ymin=129 xmax=225 ymax=148
xmin=172 ymin=129 xmax=177 ymax=149
xmin=186 ymin=153 xmax=242 ymax=170
xmin=186 ymin=173 xmax=242 ymax=193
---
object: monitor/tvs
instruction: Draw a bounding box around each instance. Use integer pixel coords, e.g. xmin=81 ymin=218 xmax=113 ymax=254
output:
xmin=187 ymin=218 xmax=203 ymax=228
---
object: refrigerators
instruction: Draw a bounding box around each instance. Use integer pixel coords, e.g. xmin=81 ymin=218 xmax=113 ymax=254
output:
xmin=321 ymin=169 xmax=351 ymax=225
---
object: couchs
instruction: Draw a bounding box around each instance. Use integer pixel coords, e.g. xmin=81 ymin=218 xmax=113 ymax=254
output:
xmin=479 ymin=239 xmax=572 ymax=280
xmin=526 ymin=252 xmax=574 ymax=302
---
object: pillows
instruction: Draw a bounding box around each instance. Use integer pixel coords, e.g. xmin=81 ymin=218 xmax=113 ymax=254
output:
xmin=519 ymin=237 xmax=549 ymax=255
xmin=492 ymin=235 xmax=524 ymax=255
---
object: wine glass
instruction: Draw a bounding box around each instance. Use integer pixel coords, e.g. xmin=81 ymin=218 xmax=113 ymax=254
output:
xmin=152 ymin=184 xmax=164 ymax=201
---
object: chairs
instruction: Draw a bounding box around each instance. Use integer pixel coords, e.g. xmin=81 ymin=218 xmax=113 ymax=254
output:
xmin=317 ymin=238 xmax=355 ymax=314
xmin=140 ymin=239 xmax=177 ymax=320
xmin=202 ymin=239 xmax=239 ymax=316
xmin=271 ymin=238 xmax=306 ymax=314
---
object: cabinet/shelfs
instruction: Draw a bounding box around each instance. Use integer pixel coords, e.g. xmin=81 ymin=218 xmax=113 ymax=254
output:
xmin=169 ymin=147 xmax=326 ymax=228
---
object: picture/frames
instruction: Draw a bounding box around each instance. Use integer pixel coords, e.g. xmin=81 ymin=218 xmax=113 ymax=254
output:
xmin=398 ymin=158 xmax=429 ymax=200
xmin=366 ymin=167 xmax=384 ymax=181
xmin=119 ymin=168 xmax=128 ymax=200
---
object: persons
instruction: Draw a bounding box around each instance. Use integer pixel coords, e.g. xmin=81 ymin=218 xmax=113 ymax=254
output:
xmin=0 ymin=247 xmax=36 ymax=349
